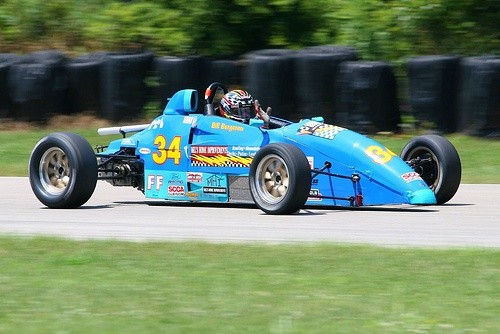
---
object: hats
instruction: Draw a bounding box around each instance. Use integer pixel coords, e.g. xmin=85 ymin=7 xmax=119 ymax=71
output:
xmin=254 ymin=100 xmax=271 ymax=128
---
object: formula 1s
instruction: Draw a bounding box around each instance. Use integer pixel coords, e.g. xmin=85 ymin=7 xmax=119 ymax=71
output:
xmin=28 ymin=82 xmax=463 ymax=216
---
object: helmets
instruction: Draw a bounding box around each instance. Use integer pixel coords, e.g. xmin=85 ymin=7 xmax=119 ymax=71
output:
xmin=219 ymin=89 xmax=257 ymax=123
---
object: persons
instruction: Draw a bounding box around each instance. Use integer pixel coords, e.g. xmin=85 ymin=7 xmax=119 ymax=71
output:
xmin=219 ymin=89 xmax=272 ymax=130
